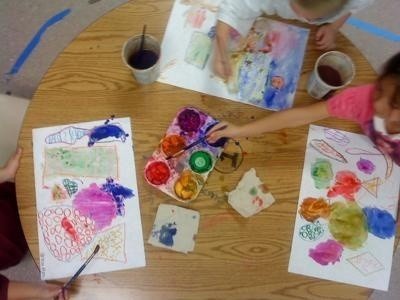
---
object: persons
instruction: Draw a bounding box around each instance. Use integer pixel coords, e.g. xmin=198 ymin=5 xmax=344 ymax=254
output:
xmin=212 ymin=0 xmax=375 ymax=82
xmin=205 ymin=52 xmax=400 ymax=168
xmin=0 ymin=146 xmax=70 ymax=300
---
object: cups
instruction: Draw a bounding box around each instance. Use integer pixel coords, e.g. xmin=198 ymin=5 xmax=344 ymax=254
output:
xmin=307 ymin=50 xmax=356 ymax=101
xmin=121 ymin=34 xmax=160 ymax=84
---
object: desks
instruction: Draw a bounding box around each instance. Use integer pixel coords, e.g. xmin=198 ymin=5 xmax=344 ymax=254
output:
xmin=14 ymin=0 xmax=400 ymax=300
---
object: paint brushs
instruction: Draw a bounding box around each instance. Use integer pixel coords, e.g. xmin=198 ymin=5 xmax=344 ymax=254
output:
xmin=166 ymin=136 xmax=209 ymax=160
xmin=62 ymin=244 xmax=101 ymax=289
xmin=138 ymin=25 xmax=147 ymax=60
